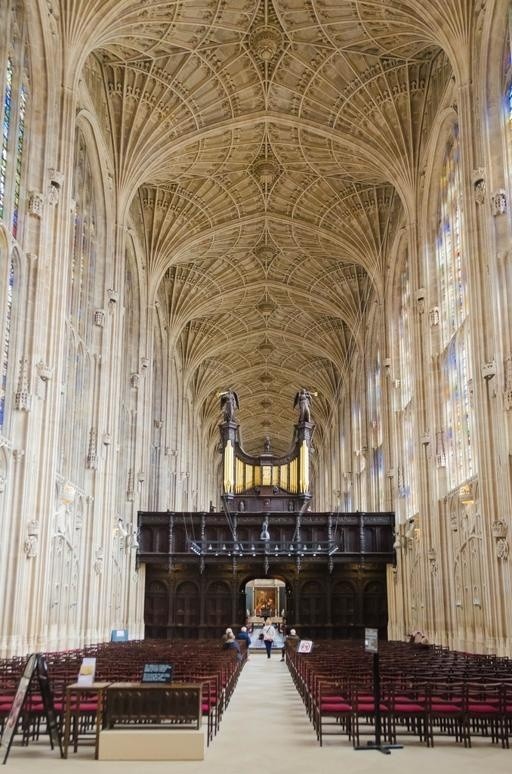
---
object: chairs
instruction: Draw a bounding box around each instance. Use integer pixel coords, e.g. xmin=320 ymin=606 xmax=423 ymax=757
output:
xmin=285 ymin=636 xmax=512 ymax=749
xmin=0 ymin=637 xmax=249 ymax=746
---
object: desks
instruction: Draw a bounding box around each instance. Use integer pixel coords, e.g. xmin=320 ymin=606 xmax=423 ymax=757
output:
xmin=59 ymin=681 xmax=208 ymax=762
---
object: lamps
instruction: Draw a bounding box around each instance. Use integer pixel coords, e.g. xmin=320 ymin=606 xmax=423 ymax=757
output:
xmin=392 ymin=523 xmax=420 ymax=548
xmin=115 ymin=518 xmax=140 ymax=549
xmin=207 ymin=543 xmax=322 ymax=558
xmin=460 ymin=483 xmax=475 ymax=504
xmin=260 ymin=522 xmax=270 ymax=540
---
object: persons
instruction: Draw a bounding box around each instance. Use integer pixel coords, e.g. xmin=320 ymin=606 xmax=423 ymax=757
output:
xmin=221 ymin=387 xmax=236 ymax=422
xmin=238 ymin=626 xmax=251 ymax=648
xmin=421 ymin=635 xmax=429 ymax=644
xmin=224 ymin=633 xmax=243 ymax=661
xmin=414 ymin=631 xmax=421 ymax=643
xmin=279 ymin=628 xmax=300 ymax=661
xmin=296 ymin=388 xmax=312 ymax=423
xmin=222 ymin=628 xmax=236 ymax=642
xmin=262 ymin=618 xmax=274 ymax=657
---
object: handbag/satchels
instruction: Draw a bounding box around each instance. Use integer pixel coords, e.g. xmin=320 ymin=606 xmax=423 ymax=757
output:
xmin=226 ymin=628 xmax=232 ymax=633
xmin=241 ymin=627 xmax=247 ymax=632
xmin=259 ymin=634 xmax=264 ymax=639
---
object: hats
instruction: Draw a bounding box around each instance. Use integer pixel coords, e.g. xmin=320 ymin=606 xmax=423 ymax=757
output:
xmin=291 ymin=629 xmax=296 ymax=635
